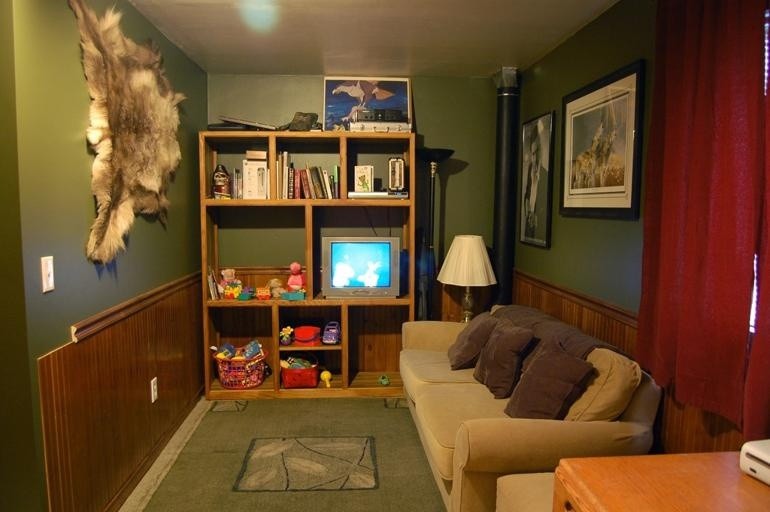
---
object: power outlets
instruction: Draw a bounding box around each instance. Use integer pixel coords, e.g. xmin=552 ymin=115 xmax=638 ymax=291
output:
xmin=150 ymin=377 xmax=159 ymax=404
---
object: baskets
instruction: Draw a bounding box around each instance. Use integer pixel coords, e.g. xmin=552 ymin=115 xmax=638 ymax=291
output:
xmin=212 ymin=346 xmax=269 ymax=390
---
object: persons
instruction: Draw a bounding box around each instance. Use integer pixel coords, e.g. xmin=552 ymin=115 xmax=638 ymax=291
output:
xmin=211 ymin=163 xmax=232 ymax=200
xmin=525 ymin=123 xmax=550 ymax=241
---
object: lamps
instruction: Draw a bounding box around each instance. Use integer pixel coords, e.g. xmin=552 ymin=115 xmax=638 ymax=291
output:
xmin=437 ymin=235 xmax=498 ymax=323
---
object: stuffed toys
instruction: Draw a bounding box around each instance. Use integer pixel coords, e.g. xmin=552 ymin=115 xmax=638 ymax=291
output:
xmin=287 ymin=262 xmax=307 ymax=293
xmin=218 ymin=269 xmax=242 ymax=294
xmin=266 ymin=277 xmax=289 ymax=297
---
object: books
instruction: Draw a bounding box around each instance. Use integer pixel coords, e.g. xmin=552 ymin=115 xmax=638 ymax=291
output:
xmin=229 ymin=147 xmax=340 ymax=200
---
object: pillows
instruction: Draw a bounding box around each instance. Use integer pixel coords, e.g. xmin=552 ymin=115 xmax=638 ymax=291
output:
xmin=447 ymin=309 xmax=498 ymax=371
xmin=473 ymin=318 xmax=534 ymax=399
xmin=495 ymin=306 xmax=642 ymax=423
xmin=504 ymin=335 xmax=593 ymax=420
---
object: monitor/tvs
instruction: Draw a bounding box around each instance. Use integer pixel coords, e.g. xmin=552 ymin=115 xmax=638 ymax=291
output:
xmin=320 ymin=236 xmax=401 ymax=299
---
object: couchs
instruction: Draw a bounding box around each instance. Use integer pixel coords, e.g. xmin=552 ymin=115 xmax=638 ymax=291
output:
xmin=399 ymin=304 xmax=663 ymax=512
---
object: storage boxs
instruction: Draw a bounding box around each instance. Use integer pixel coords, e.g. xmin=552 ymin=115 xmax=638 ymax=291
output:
xmin=281 ymin=364 xmax=319 ymax=389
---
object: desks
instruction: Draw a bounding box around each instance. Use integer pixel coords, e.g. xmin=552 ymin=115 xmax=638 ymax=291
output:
xmin=544 ymin=448 xmax=770 ymax=512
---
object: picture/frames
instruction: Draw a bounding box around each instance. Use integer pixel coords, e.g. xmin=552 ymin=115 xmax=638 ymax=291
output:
xmin=322 ymin=77 xmax=412 ymax=131
xmin=556 ymin=58 xmax=644 ymax=221
xmin=520 ymin=109 xmax=555 ymax=249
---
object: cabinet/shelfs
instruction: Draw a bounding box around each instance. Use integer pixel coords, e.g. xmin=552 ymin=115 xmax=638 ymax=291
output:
xmin=199 ymin=131 xmax=415 ymax=400
